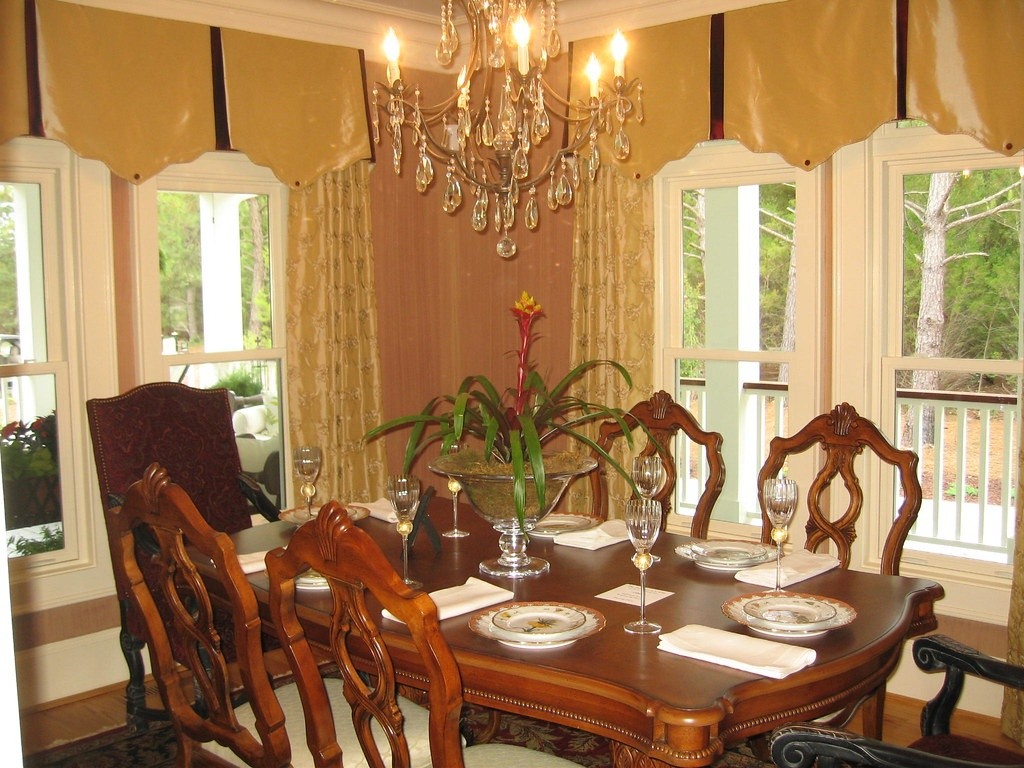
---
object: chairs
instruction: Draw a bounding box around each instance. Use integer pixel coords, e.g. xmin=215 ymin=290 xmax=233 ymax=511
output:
xmin=768 ymin=633 xmax=1024 ymax=767
xmin=589 ymin=389 xmax=726 ymax=542
xmin=264 ymin=500 xmax=592 ymax=768
xmin=757 ymin=402 xmax=922 ymax=741
xmin=85 ymin=381 xmax=340 ymax=736
xmin=105 ymin=461 xmax=466 ymax=768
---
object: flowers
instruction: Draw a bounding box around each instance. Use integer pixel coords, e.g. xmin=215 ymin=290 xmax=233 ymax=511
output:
xmin=361 ymin=290 xmax=669 ymax=543
xmin=0 ymin=409 xmax=65 ymax=557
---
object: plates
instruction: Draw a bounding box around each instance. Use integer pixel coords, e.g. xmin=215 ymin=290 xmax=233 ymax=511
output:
xmin=278 ymin=505 xmax=371 ymax=524
xmin=743 ymin=598 xmax=837 ymax=623
xmin=691 ymin=541 xmax=766 ymax=559
xmin=468 ymin=601 xmax=606 ymax=649
xmin=492 ymin=606 xmax=586 ymax=634
xmin=720 ymin=592 xmax=858 ymax=637
xmin=263 ymin=569 xmax=328 ymax=589
xmin=674 ymin=539 xmax=784 ymax=570
xmin=527 ymin=512 xmax=604 ymax=537
xmin=534 ymin=516 xmax=591 ymax=531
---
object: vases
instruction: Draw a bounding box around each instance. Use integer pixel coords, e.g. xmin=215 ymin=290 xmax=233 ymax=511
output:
xmin=428 ymin=449 xmax=597 ymax=577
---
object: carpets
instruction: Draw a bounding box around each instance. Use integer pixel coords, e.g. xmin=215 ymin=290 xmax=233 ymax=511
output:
xmin=22 ymin=661 xmax=778 ymax=768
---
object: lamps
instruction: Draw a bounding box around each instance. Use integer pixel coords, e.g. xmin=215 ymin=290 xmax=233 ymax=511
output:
xmin=372 ymin=0 xmax=644 ymax=257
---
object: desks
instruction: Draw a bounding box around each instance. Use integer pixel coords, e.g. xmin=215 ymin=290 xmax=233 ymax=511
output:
xmin=182 ymin=495 xmax=945 ymax=768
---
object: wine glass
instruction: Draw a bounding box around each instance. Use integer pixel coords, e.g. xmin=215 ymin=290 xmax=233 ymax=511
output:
xmin=293 ymin=446 xmax=321 ymax=519
xmin=632 ymin=457 xmax=662 ymax=562
xmin=387 ymin=475 xmax=423 ymax=589
xmin=441 ymin=443 xmax=469 ymax=537
xmin=624 ymin=500 xmax=662 ymax=634
xmin=763 ymin=480 xmax=798 ymax=593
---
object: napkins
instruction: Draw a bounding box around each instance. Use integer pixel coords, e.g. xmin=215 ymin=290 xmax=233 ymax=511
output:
xmin=734 ymin=548 xmax=841 ymax=588
xmin=346 ymin=498 xmax=400 ymax=523
xmin=381 ymin=576 xmax=515 ymax=626
xmin=236 ymin=546 xmax=289 ymax=574
xmin=553 ymin=520 xmax=631 ymax=550
xmin=656 ymin=624 xmax=817 ymax=680
